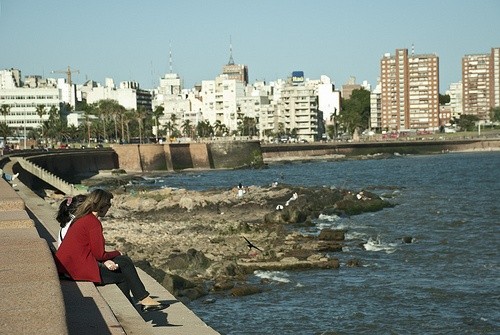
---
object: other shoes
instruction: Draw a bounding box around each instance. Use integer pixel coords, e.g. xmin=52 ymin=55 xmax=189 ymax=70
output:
xmin=12 ymin=184 xmax=17 ymax=187
xmin=141 ymin=303 xmax=170 ymax=314
xmin=12 ymin=173 xmax=19 ymax=180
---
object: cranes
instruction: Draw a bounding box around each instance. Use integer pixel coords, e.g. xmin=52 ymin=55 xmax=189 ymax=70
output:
xmin=49 ymin=65 xmax=81 ymax=85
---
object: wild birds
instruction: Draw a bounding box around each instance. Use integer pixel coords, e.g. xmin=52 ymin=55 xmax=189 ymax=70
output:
xmin=243 ymin=236 xmax=263 ymax=253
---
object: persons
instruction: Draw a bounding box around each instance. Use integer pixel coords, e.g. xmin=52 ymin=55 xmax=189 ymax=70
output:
xmin=244 ymin=185 xmax=250 ymax=194
xmin=57 ymin=192 xmax=92 ymax=247
xmin=56 ymin=190 xmax=170 ymax=312
xmin=0 ymin=140 xmax=6 ymax=155
xmin=238 ymin=183 xmax=243 ymax=196
xmin=0 ymin=169 xmax=19 ymax=188
